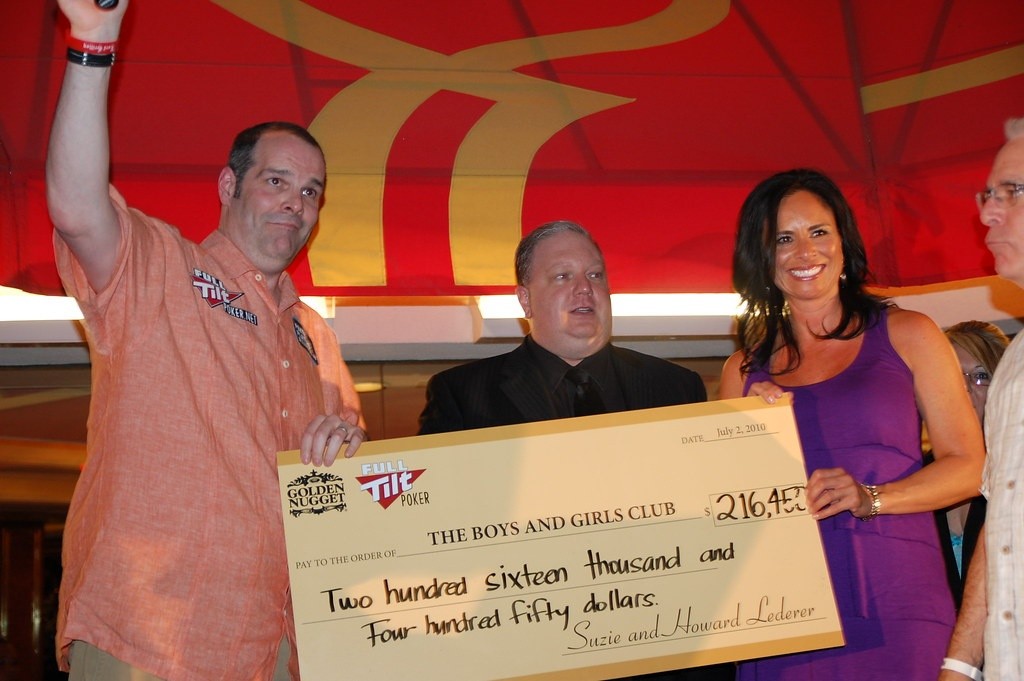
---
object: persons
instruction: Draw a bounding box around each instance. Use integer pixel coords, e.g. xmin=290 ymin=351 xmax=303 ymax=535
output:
xmin=416 ymin=222 xmax=738 ymax=681
xmin=721 ymin=168 xmax=986 ymax=681
xmin=46 ymin=0 xmax=369 ymax=681
xmin=924 ymin=116 xmax=1024 ymax=681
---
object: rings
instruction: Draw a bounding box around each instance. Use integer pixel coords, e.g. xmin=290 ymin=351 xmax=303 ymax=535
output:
xmin=337 ymin=427 xmax=348 ymax=435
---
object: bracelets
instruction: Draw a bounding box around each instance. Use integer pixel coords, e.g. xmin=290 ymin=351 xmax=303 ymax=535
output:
xmin=941 ymin=658 xmax=983 ymax=681
xmin=856 ymin=485 xmax=882 ymax=522
xmin=67 ymin=35 xmax=118 ymax=54
xmin=67 ymin=48 xmax=116 ymax=67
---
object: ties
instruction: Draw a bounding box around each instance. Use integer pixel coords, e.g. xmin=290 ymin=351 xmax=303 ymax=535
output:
xmin=564 ymin=367 xmax=607 ymax=416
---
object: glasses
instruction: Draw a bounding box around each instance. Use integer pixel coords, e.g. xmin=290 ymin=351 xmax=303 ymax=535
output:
xmin=974 ymin=183 xmax=1024 ymax=210
xmin=962 ymin=370 xmax=993 ymax=386
